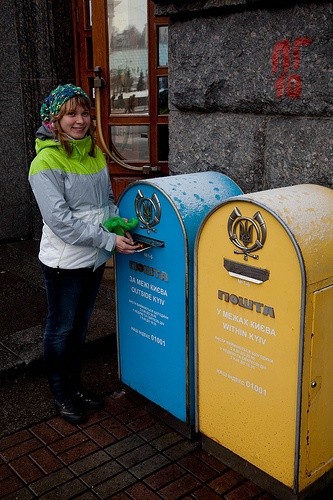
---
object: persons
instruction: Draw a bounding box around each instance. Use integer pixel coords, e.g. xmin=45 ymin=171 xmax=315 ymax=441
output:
xmin=157 ymin=78 xmax=169 ymax=160
xmin=110 ymin=93 xmax=136 ymax=113
xmin=28 ymin=82 xmax=143 ymax=424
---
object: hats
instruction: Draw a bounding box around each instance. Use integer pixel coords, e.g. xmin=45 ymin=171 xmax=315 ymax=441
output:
xmin=39 ymin=82 xmax=89 ymax=120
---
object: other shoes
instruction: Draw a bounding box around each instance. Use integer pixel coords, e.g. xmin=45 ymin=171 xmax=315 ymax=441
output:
xmin=55 ymin=389 xmax=103 ymax=424
